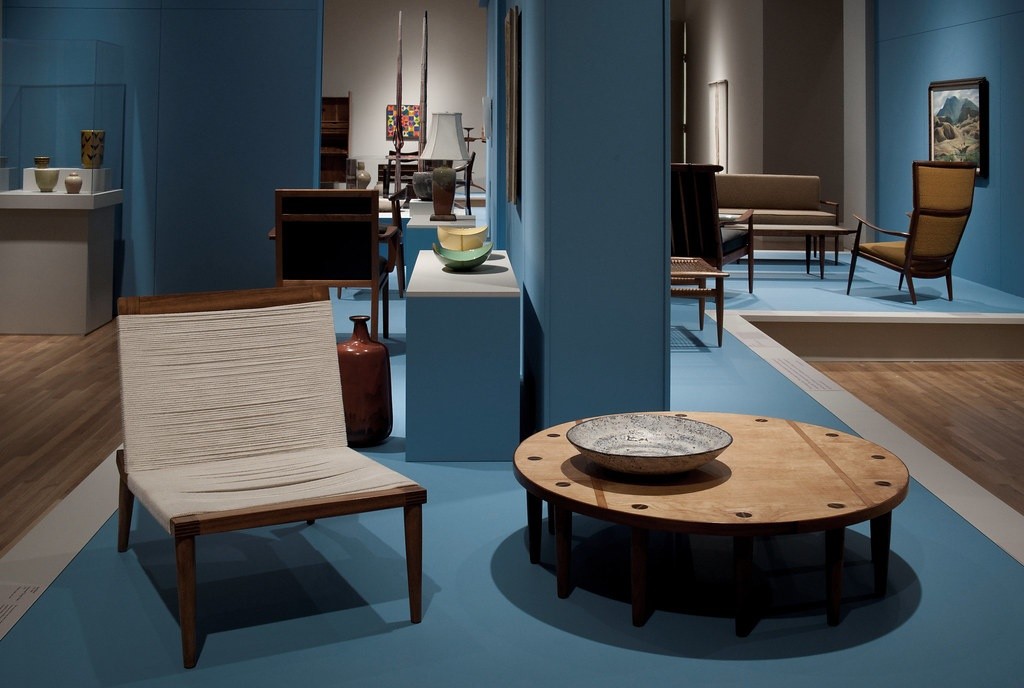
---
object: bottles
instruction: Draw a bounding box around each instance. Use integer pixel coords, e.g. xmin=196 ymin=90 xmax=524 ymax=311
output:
xmin=65 ymin=173 xmax=83 ymax=194
xmin=356 ymin=161 xmax=371 ymax=189
xmin=337 ymin=316 xmax=394 ymax=448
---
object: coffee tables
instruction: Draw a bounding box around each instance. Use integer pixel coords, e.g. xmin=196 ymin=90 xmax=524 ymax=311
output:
xmin=725 ymin=223 xmax=857 ymax=280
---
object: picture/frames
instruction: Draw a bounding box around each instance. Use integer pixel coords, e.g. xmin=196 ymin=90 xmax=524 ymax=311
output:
xmin=504 ymin=5 xmax=518 ymax=204
xmin=927 ymin=77 xmax=989 ymax=179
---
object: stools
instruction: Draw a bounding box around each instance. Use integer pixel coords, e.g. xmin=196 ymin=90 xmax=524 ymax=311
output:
xmin=670 ymin=257 xmax=730 ymax=347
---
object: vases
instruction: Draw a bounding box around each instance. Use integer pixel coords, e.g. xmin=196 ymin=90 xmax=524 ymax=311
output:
xmin=337 ymin=315 xmax=393 ymax=448
xmin=357 ymin=162 xmax=371 ymax=188
xmin=413 ymin=172 xmax=434 ymax=201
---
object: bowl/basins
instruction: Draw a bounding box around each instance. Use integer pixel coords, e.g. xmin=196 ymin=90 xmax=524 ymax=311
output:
xmin=566 ymin=414 xmax=733 ymax=483
xmin=34 ymin=157 xmax=50 ymax=168
xmin=34 ymin=169 xmax=60 ymax=192
xmin=413 ymin=172 xmax=433 ymax=200
xmin=0 ymin=156 xmax=8 ymax=168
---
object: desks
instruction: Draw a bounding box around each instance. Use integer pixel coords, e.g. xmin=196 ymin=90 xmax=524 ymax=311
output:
xmin=511 ymin=412 xmax=910 ymax=640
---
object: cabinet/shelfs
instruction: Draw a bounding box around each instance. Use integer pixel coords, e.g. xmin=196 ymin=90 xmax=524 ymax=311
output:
xmin=320 ymin=90 xmax=352 ymax=184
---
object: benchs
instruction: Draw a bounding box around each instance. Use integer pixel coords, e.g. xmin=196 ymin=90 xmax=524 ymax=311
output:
xmin=714 ymin=174 xmax=840 ymax=264
xmin=375 ymin=164 xmax=418 ymax=195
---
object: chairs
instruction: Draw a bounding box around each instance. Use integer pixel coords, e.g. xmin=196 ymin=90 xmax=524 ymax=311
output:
xmin=847 ymin=160 xmax=978 ymax=305
xmin=115 ymin=282 xmax=427 ymax=668
xmin=274 ymin=188 xmax=400 ymax=342
xmin=383 ymin=150 xmax=418 ymax=199
xmin=669 ymin=163 xmax=754 ymax=302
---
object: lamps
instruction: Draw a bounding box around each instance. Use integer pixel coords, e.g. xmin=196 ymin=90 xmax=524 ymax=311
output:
xmin=420 ymin=111 xmax=472 ymax=220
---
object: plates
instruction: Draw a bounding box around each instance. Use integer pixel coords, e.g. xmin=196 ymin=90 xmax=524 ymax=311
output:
xmin=432 ymin=243 xmax=494 ymax=270
xmin=437 ymin=226 xmax=488 ymax=251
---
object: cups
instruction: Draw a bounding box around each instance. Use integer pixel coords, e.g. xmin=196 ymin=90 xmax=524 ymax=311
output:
xmin=81 ymin=129 xmax=106 ymax=169
